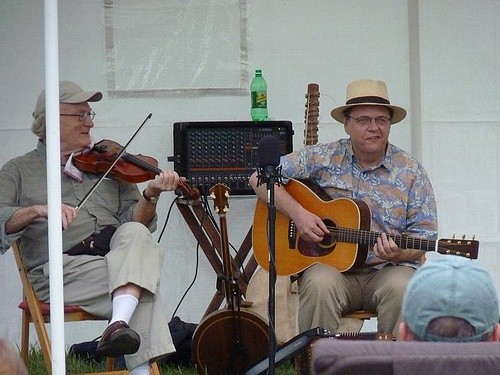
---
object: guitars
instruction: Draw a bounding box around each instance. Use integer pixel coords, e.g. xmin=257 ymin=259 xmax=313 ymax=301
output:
xmin=193 ymin=182 xmax=278 ymax=375
xmin=251 ymin=178 xmax=479 ymax=276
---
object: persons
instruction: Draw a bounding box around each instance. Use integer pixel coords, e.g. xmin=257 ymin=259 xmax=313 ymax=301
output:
xmin=0 ymin=80 xmax=186 ymax=375
xmin=250 ymin=80 xmax=438 ymax=333
xmin=398 ymin=255 xmax=500 ymax=342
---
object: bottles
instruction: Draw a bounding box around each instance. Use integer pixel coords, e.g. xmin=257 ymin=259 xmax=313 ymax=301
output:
xmin=250 ymin=69 xmax=269 ymax=122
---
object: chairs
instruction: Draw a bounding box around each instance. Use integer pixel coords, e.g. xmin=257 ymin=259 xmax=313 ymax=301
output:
xmin=11 ymin=240 xmax=163 ymax=375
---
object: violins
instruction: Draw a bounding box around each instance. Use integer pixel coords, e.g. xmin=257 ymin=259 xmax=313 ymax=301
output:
xmin=70 ymin=138 xmax=201 ymax=202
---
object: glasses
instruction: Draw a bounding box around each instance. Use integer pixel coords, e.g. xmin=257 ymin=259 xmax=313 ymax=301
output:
xmin=347 ymin=114 xmax=392 ymax=126
xmin=60 ymin=110 xmax=96 ymax=119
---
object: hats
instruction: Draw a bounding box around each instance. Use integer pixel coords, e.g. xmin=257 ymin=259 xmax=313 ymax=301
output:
xmin=330 ymin=79 xmax=407 ymax=124
xmin=32 ymin=80 xmax=103 ymax=120
xmin=401 ymin=255 xmax=500 ymax=343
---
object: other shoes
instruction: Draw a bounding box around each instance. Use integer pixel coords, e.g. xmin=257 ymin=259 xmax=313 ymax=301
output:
xmin=96 ymin=320 xmax=140 ymax=357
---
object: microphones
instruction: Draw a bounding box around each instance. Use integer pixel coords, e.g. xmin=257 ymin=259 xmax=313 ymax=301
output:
xmin=258 ymin=135 xmax=279 ymax=179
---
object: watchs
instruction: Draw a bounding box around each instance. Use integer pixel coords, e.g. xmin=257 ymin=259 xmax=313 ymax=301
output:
xmin=142 ymin=189 xmax=158 ymax=203
